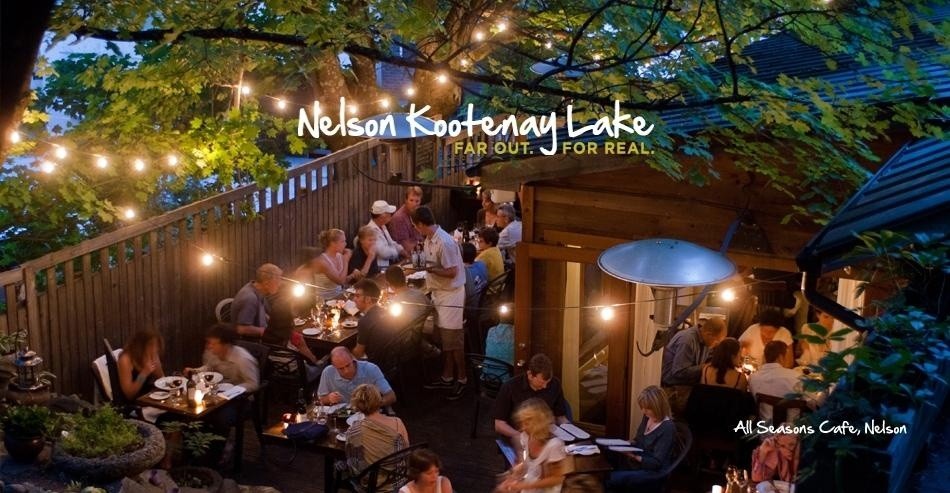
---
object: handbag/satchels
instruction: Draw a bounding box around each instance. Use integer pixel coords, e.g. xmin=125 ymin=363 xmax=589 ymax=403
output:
xmin=287 ymin=421 xmax=330 ymax=447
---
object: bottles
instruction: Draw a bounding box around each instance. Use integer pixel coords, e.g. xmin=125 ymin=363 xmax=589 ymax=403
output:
xmin=316 ymin=397 xmax=329 ymax=425
xmin=296 ymin=387 xmax=308 ymax=423
xmin=282 ymin=412 xmax=293 ymax=434
xmin=329 ymin=417 xmax=340 ymax=444
xmin=453 ymin=220 xmax=470 ymax=244
xmin=742 ymin=356 xmax=754 ymax=382
xmin=414 ymin=238 xmax=423 ymax=267
xmin=186 ymin=368 xmax=198 ymax=406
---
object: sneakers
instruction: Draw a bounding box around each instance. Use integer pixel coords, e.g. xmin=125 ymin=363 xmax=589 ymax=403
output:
xmin=447 ymin=378 xmax=469 ymax=400
xmin=424 ymin=378 xmax=456 ymax=389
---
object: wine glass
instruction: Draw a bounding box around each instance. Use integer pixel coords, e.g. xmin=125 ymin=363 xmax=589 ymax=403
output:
xmin=311 ymin=390 xmax=321 ymax=408
xmin=725 ymin=464 xmax=750 ymax=493
xmin=203 ymin=367 xmax=215 ymax=396
xmin=306 ymin=283 xmax=355 ymax=334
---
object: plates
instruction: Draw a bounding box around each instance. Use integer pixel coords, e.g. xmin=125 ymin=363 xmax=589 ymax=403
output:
xmin=292 ymin=297 xmax=358 ymax=336
xmin=150 ymin=370 xmax=235 ymax=400
xmin=756 ymin=477 xmax=795 ymax=493
xmin=313 ymin=405 xmax=331 ymax=415
xmin=336 ymin=431 xmax=348 ymax=442
xmin=566 ymin=444 xmax=601 ymax=455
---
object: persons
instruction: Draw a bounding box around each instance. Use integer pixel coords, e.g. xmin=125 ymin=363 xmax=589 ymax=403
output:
xmin=313 ymin=344 xmax=397 ymax=410
xmin=181 ymin=322 xmax=261 ymax=423
xmin=113 ymin=324 xmax=169 ymax=399
xmin=109 ymin=182 xmax=837 ymax=493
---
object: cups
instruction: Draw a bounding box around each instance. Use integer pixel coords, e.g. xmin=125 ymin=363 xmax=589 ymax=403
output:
xmin=410 ymin=250 xmax=427 ymax=268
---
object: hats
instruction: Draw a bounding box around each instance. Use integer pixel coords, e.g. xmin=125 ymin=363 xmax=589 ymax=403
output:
xmin=370 ymin=200 xmax=396 ymax=215
